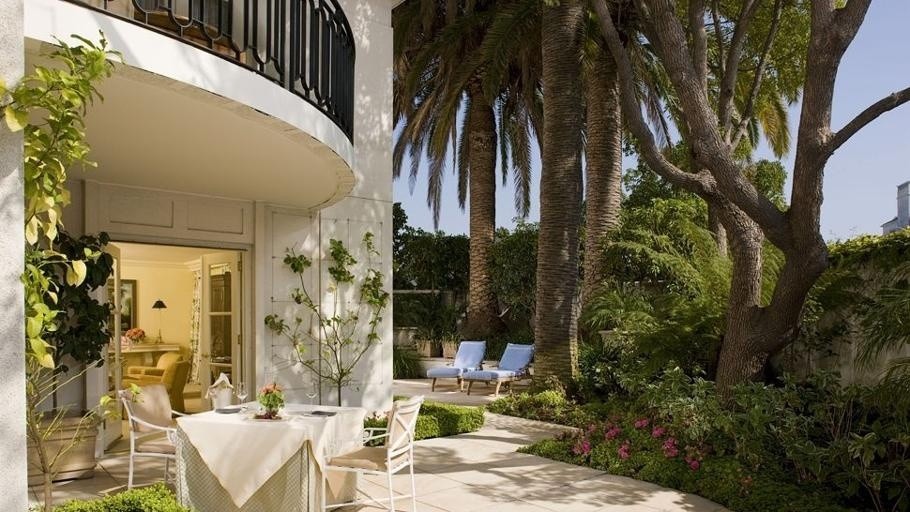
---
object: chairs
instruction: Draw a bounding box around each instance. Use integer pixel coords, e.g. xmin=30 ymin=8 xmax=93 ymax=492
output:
xmin=118 ymin=351 xmax=196 ymax=490
xmin=320 ymin=393 xmax=426 ymax=511
xmin=426 ymin=340 xmax=535 ymax=396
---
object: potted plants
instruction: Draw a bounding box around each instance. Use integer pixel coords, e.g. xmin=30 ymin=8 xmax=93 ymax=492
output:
xmin=28 ymin=222 xmax=116 ymax=489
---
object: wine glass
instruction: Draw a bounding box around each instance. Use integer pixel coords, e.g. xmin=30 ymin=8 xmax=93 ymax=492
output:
xmin=236 ymin=382 xmax=247 ymax=410
xmin=304 ymin=382 xmax=317 ymax=410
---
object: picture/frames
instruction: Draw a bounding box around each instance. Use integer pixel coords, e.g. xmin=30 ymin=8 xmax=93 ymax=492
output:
xmin=105 ymin=279 xmax=137 ymax=335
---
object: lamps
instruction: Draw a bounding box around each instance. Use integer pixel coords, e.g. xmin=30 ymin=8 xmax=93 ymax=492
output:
xmin=151 ymin=298 xmax=167 ymax=343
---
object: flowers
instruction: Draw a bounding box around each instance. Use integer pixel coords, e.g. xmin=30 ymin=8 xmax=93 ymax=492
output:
xmin=125 ymin=328 xmax=146 ymax=343
xmin=256 ymin=381 xmax=285 ymax=415
